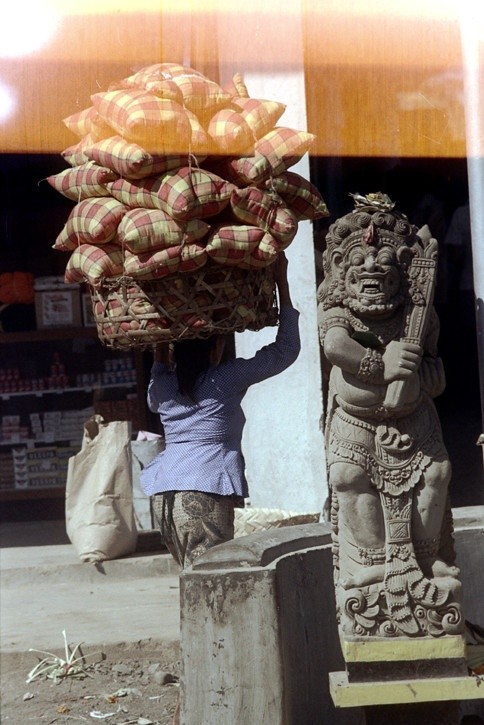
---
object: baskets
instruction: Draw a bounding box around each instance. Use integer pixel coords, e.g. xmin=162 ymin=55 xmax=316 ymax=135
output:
xmin=91 ymin=251 xmax=278 ymax=351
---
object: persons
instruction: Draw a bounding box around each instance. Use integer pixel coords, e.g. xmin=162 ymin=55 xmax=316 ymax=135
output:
xmin=315 ymin=203 xmax=461 ymax=591
xmin=144 ymin=247 xmax=302 ymax=568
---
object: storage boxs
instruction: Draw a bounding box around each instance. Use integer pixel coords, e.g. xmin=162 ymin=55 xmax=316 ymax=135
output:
xmin=35 ymin=285 xmax=82 ymax=328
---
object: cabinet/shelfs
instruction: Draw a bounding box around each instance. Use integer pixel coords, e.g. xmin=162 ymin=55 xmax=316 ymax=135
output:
xmin=0 ymin=382 xmax=137 ymax=445
